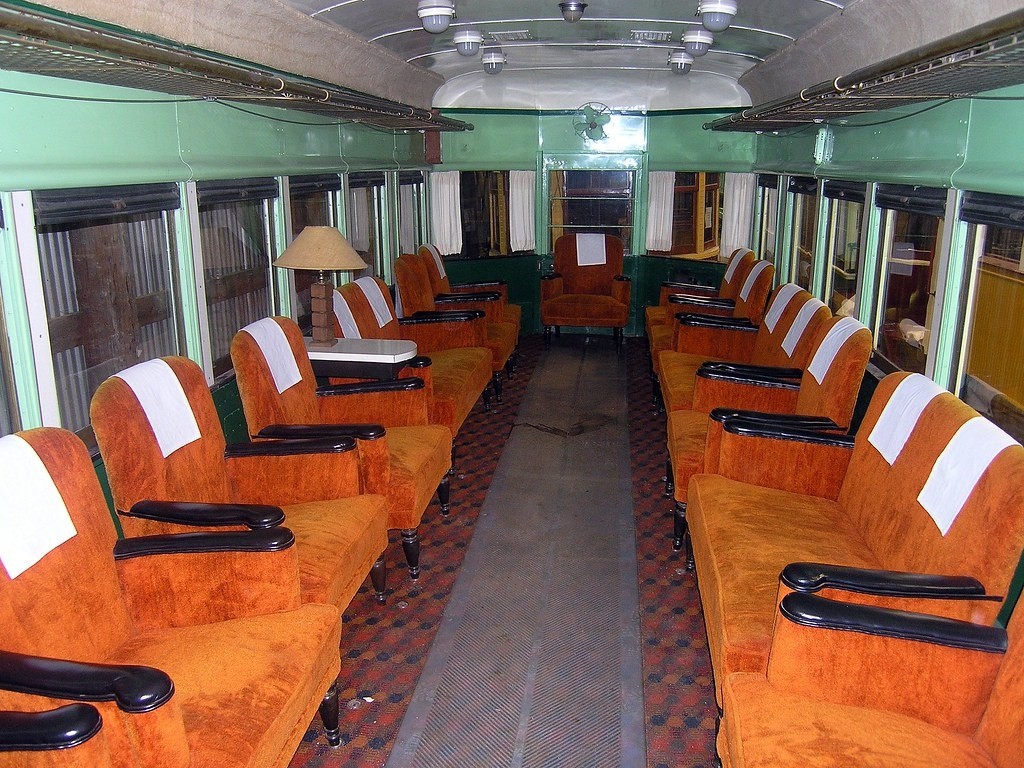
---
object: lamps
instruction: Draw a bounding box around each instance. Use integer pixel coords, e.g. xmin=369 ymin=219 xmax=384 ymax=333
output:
xmin=667 ymin=50 xmax=695 ymax=75
xmin=272 ymin=225 xmax=369 ymax=347
xmin=558 ymin=3 xmax=588 ymax=23
xmin=481 ymin=53 xmax=508 ymax=74
xmin=694 ymin=0 xmax=739 ymax=32
xmin=417 ymin=0 xmax=458 ymax=34
xmin=679 ymin=25 xmax=714 ymax=57
xmin=453 ymin=30 xmax=485 ymax=56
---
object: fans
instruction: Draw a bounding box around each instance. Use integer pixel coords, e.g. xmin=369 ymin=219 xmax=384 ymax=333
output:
xmin=572 ymin=102 xmax=615 ymax=142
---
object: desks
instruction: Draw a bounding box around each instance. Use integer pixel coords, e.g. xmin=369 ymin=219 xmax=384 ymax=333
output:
xmin=303 ymin=335 xmax=419 ymax=384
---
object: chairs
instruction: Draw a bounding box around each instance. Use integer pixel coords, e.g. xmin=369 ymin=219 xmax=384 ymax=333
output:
xmin=0 ymin=232 xmax=1024 ymax=768
